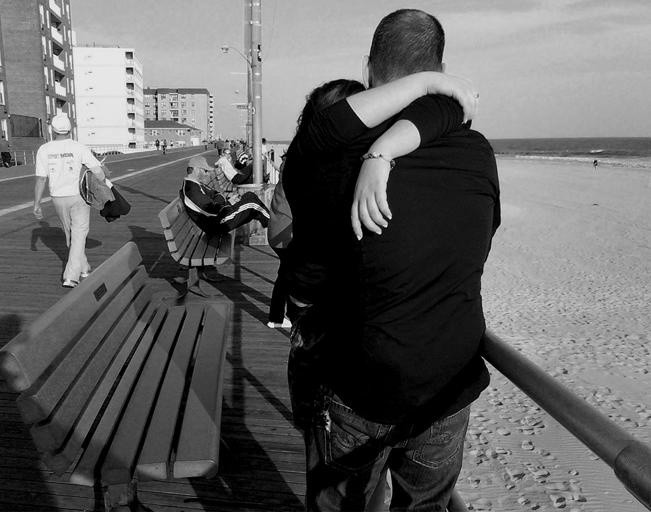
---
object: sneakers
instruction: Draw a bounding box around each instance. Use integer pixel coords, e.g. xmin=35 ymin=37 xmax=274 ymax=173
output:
xmin=63 ymin=270 xmax=88 ymax=288
xmin=267 ymin=318 xmax=294 ymax=328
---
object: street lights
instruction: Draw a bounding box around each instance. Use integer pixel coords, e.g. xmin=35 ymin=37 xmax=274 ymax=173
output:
xmin=222 ymin=44 xmax=263 ymax=183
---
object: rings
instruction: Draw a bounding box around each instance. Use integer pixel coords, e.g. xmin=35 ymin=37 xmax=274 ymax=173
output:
xmin=475 ymin=92 xmax=480 ymax=99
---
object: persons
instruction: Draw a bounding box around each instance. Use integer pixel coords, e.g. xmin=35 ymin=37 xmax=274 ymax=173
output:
xmin=289 ymin=9 xmax=500 ymax=511
xmin=0 ymin=136 xmax=11 ymax=168
xmin=179 ymin=137 xmax=277 ymax=236
xmin=155 ymin=137 xmax=173 ymax=155
xmin=33 ymin=115 xmax=106 ymax=286
xmin=271 ymin=69 xmax=480 ymax=424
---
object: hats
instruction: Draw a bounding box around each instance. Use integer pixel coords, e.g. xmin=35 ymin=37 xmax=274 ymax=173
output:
xmin=188 ymin=156 xmax=214 ymax=171
xmin=51 ymin=116 xmax=71 ymax=134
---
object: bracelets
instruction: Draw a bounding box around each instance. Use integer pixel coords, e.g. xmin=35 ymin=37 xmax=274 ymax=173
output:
xmin=360 ymin=152 xmax=396 ymax=169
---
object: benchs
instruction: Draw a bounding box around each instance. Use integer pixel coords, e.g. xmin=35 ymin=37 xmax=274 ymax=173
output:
xmin=158 ymin=171 xmax=234 ymax=298
xmin=0 ymin=242 xmax=232 ymax=512
xmin=214 ymin=167 xmax=237 ymax=192
